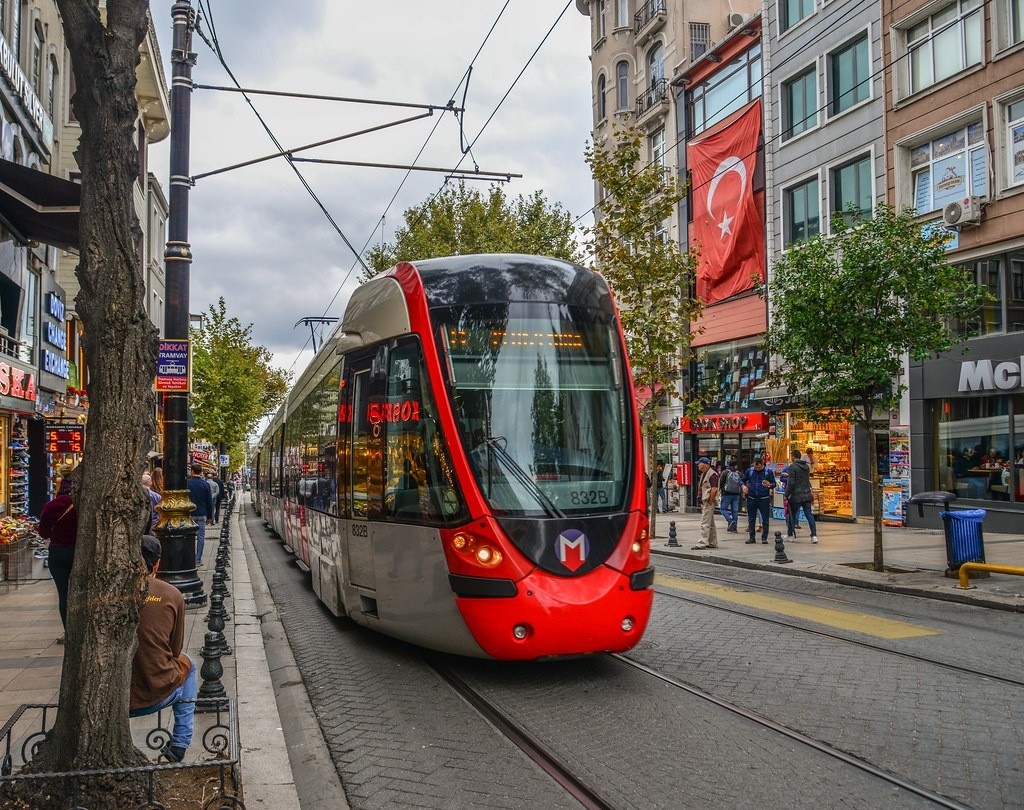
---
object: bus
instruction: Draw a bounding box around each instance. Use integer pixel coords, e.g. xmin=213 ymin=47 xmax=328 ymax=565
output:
xmin=248 ymin=252 xmax=655 ymax=662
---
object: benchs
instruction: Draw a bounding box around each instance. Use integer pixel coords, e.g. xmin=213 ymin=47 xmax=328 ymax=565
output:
xmin=990 ymin=463 xmax=1024 ymax=500
xmin=948 ymin=466 xmax=978 ymax=500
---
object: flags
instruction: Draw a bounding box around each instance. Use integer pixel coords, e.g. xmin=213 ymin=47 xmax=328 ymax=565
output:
xmin=686 ymin=99 xmax=766 ymax=305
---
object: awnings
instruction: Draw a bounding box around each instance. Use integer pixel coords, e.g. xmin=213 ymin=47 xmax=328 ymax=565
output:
xmin=634 ymin=383 xmax=663 ymax=408
xmin=0 ymin=158 xmax=82 ymax=255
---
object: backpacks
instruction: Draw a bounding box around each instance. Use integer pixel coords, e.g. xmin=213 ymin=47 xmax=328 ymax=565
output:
xmin=724 ymin=469 xmax=741 ymax=494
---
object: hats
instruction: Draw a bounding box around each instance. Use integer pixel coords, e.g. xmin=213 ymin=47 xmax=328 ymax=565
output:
xmin=140 ymin=535 xmax=162 ymax=558
xmin=695 ymin=457 xmax=710 ymax=465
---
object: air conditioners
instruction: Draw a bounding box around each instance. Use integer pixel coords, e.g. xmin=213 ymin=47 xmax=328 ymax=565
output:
xmin=727 ymin=12 xmax=750 ymax=29
xmin=942 ymin=195 xmax=981 ymax=231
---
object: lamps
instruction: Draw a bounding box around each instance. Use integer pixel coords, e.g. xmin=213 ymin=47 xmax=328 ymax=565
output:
xmin=705 ymin=55 xmax=723 ymax=62
xmin=740 ymin=29 xmax=758 ymax=36
xmin=677 ymin=79 xmax=692 ymax=86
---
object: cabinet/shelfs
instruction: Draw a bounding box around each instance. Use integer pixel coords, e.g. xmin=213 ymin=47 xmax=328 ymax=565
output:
xmin=6 ymin=426 xmax=30 ymax=517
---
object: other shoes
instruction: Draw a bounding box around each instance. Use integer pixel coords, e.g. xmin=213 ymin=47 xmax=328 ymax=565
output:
xmin=691 ymin=546 xmax=702 ymax=550
xmin=781 ymin=534 xmax=794 ymax=541
xmin=756 ymin=526 xmax=763 ymax=532
xmin=196 ymin=562 xmax=204 ymax=567
xmin=216 ymin=520 xmax=220 ymax=523
xmin=656 ymin=510 xmax=660 ymax=513
xmin=762 ymin=537 xmax=767 ymax=544
xmin=160 ymin=740 xmax=185 ymax=764
xmin=795 ymin=524 xmax=801 ymax=529
xmin=744 ymin=527 xmax=750 ymax=532
xmin=727 ymin=520 xmax=738 ymax=533
xmin=706 ymin=544 xmax=718 ymax=548
xmin=662 ymin=510 xmax=667 ymax=514
xmin=745 ymin=538 xmax=756 ymax=544
xmin=210 ymin=521 xmax=215 ymax=526
xmin=810 ymin=536 xmax=818 ymax=542
xmin=56 ymin=633 xmax=65 ymax=642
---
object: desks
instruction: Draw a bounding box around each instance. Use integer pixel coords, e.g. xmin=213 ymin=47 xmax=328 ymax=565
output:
xmin=968 ymin=469 xmax=1002 ymax=499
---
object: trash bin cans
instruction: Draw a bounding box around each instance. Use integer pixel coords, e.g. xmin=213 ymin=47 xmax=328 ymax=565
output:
xmin=942 ymin=510 xmax=985 ymax=578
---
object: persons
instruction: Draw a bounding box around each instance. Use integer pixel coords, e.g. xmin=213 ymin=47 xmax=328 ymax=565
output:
xmin=39 ymin=479 xmax=76 ymax=643
xmin=719 ymin=460 xmax=745 ymax=532
xmin=691 ymin=457 xmax=720 ymax=550
xmin=142 ymin=467 xmax=164 ymax=531
xmin=129 ymin=535 xmax=196 ymax=763
xmin=739 ymin=458 xmax=777 ymax=543
xmin=206 ymin=471 xmax=224 ymax=525
xmin=801 ymin=448 xmax=819 ymax=468
xmin=645 ymin=461 xmax=666 ymax=514
xmin=188 ymin=464 xmax=215 ymax=567
xmin=780 ymin=450 xmax=818 ymax=543
xmin=954 ymin=446 xmax=1024 ymax=500
